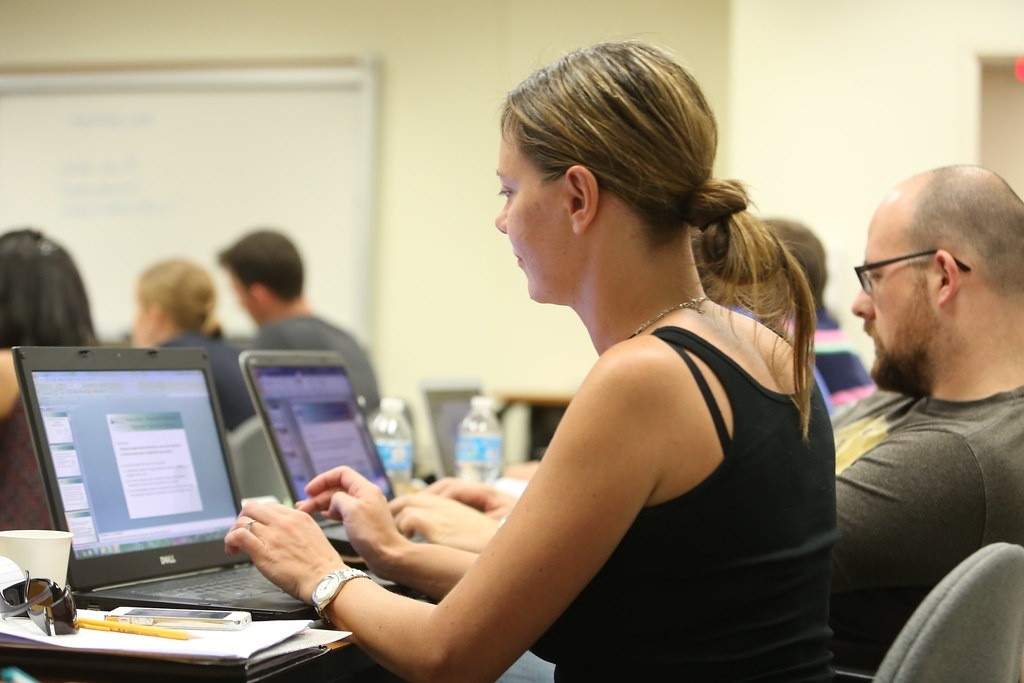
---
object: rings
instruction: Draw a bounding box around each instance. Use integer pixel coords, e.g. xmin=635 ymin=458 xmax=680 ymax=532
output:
xmin=248 ymin=520 xmax=256 ymax=531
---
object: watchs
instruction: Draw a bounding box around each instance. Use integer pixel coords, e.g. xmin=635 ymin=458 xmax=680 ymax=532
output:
xmin=311 ymin=569 xmax=371 ymax=626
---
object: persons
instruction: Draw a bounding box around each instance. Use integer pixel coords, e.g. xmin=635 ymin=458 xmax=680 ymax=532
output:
xmin=388 ymin=164 xmax=1024 ymax=683
xmin=0 ymin=232 xmax=97 ymax=532
xmin=131 ymin=259 xmax=255 ymax=431
xmin=224 ymin=38 xmax=838 ymax=683
xmin=763 ymin=218 xmax=877 ymax=412
xmin=220 ymin=231 xmax=381 ymax=408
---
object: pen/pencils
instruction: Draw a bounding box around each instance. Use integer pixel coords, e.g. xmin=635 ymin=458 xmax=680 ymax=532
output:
xmin=75 ymin=620 xmax=201 ymax=641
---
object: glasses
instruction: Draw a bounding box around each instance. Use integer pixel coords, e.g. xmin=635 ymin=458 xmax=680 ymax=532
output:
xmin=854 ymin=251 xmax=971 ymax=297
xmin=0 ymin=570 xmax=80 ymax=636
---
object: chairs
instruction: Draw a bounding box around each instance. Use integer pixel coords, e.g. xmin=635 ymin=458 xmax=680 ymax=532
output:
xmin=871 ymin=541 xmax=1024 ymax=683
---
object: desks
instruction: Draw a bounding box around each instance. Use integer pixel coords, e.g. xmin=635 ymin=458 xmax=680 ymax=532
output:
xmin=0 ymin=544 xmax=424 ymax=683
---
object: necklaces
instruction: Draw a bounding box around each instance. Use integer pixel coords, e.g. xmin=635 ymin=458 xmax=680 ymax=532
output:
xmin=628 ymin=296 xmax=709 ymax=340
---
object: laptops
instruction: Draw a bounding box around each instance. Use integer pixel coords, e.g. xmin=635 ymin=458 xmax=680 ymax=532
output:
xmin=10 ymin=340 xmax=484 ymax=625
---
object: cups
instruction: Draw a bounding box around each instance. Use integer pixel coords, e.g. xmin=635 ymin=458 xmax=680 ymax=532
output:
xmin=0 ymin=530 xmax=74 ymax=593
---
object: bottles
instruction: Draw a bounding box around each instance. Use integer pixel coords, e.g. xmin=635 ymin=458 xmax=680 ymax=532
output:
xmin=369 ymin=397 xmax=414 ymax=498
xmin=455 ymin=395 xmax=502 ymax=484
